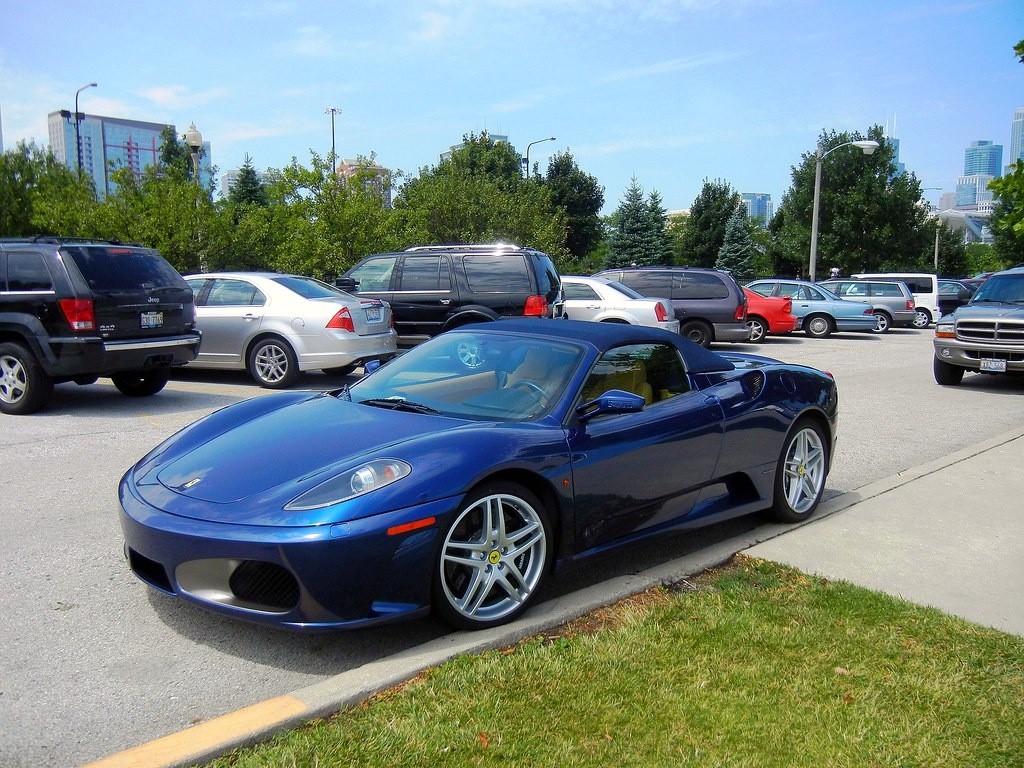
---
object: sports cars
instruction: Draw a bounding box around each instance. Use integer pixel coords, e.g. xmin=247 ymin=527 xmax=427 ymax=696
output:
xmin=118 ymin=316 xmax=837 ymax=638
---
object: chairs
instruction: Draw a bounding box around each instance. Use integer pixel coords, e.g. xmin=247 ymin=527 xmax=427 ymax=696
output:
xmin=582 ymin=358 xmax=650 ymax=419
xmin=511 ymin=351 xmax=563 ymax=408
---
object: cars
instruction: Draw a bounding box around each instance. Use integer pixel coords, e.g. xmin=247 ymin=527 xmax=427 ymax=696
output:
xmin=741 ymin=287 xmax=798 ymax=343
xmin=937 ymin=279 xmax=977 ymax=317
xmin=744 ymin=279 xmax=878 ymax=337
xmin=973 ymin=272 xmax=996 ymax=279
xmin=182 ymin=271 xmax=397 ymax=389
xmin=559 ymin=276 xmax=680 ymax=334
xmin=959 ymin=279 xmax=987 ymax=289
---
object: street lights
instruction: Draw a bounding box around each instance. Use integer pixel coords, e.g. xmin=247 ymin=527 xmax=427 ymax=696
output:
xmin=76 ymin=82 xmax=98 ymax=179
xmin=186 ymin=122 xmax=210 ymax=273
xmin=809 ymin=140 xmax=879 ymax=281
xmin=527 ymin=137 xmax=557 ymax=179
xmin=324 ymin=107 xmax=343 ymax=174
xmin=934 ymin=217 xmax=942 ymax=268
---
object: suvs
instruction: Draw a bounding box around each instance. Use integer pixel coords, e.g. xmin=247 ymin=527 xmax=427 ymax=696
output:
xmin=320 ymin=245 xmax=570 ymax=375
xmin=934 ymin=266 xmax=1024 ymax=389
xmin=1 ymin=236 xmax=203 ymax=413
xmin=791 ymin=278 xmax=917 ymax=334
xmin=589 ymin=267 xmax=750 ymax=349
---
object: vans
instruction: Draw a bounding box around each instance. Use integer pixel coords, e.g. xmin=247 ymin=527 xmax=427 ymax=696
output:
xmin=835 ymin=273 xmax=942 ymax=329
